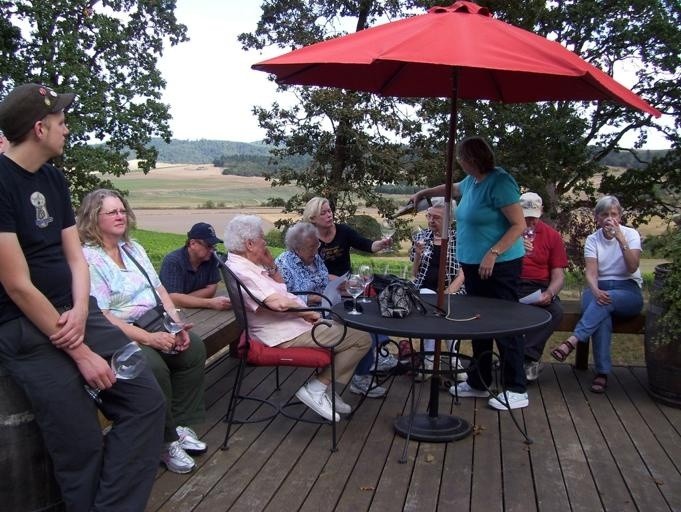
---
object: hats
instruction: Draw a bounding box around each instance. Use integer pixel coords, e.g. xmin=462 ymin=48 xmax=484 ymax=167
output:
xmin=519 ymin=192 xmax=542 ymax=218
xmin=0 ymin=83 xmax=74 ymax=141
xmin=188 ymin=223 xmax=223 ymax=244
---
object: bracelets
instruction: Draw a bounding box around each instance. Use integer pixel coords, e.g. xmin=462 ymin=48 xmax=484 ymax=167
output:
xmin=489 ymin=247 xmax=500 ymax=257
xmin=265 ymin=263 xmax=277 ymax=274
xmin=622 ymin=244 xmax=629 ymax=251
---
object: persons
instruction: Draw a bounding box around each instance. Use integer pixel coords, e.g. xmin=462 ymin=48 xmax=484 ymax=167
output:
xmin=71 ymin=185 xmax=212 ymax=475
xmin=511 ymin=192 xmax=571 ymax=382
xmin=409 ymin=200 xmax=470 ymax=383
xmin=406 ymin=135 xmax=533 ymax=411
xmin=272 ymin=220 xmax=389 ymax=398
xmin=159 ymin=221 xmax=232 ymax=310
xmin=304 ymin=195 xmax=399 ymax=399
xmin=551 ymin=193 xmax=646 ymax=393
xmin=1 ymin=81 xmax=165 ymax=512
xmin=225 ymin=214 xmax=374 ymax=424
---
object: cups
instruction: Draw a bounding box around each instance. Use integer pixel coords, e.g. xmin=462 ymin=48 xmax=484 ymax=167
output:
xmin=604 ymin=217 xmax=616 ymax=238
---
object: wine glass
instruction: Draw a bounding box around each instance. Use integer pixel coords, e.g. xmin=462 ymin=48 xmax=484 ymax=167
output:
xmin=83 ymin=340 xmax=148 ymax=402
xmin=159 ymin=306 xmax=189 ymax=356
xmin=521 ymin=226 xmax=537 ymax=256
xmin=345 ymin=264 xmax=375 ymax=315
xmin=408 ymin=226 xmax=430 ymax=256
xmin=379 ymin=218 xmax=396 ymax=253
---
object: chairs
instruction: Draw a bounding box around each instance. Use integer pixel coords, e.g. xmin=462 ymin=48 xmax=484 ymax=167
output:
xmin=213 ymin=249 xmax=348 ymax=451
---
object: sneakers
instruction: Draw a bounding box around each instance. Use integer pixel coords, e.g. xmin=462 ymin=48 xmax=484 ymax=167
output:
xmin=160 ymin=425 xmax=207 ymax=474
xmin=349 ymin=351 xmax=466 ymax=398
xmin=449 ymin=381 xmax=489 ymax=398
xmin=487 ymin=390 xmax=528 ymax=411
xmin=294 ymin=382 xmax=351 ymax=422
xmin=523 ymin=358 xmax=544 ymax=381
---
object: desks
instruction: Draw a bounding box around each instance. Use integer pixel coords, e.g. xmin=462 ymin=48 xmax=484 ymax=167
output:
xmin=330 ymin=294 xmax=552 ymax=464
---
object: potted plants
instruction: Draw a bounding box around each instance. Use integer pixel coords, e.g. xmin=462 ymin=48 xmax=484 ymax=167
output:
xmin=641 ymin=226 xmax=681 ymax=409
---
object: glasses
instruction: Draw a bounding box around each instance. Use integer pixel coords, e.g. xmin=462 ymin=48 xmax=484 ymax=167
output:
xmin=343 ymin=300 xmax=363 ymax=312
xmin=96 ymin=209 xmax=127 ymax=215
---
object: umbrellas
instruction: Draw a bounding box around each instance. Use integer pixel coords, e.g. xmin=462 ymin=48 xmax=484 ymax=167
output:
xmin=252 ymin=0 xmax=666 ymax=418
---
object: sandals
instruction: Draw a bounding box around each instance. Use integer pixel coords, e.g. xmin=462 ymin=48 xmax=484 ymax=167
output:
xmin=589 ymin=375 xmax=607 ymax=393
xmin=550 ymin=341 xmax=574 ymax=362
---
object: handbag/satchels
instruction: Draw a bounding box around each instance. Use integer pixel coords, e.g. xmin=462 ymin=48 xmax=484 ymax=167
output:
xmin=133 ymin=306 xmax=174 ymax=333
xmin=371 ymin=273 xmax=412 ymax=318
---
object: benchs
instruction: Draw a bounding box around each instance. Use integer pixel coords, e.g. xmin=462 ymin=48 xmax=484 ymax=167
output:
xmin=172 ymin=306 xmax=242 ymax=359
xmin=546 ymin=300 xmax=647 ymax=370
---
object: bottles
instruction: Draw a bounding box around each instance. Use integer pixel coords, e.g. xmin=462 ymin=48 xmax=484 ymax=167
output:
xmin=387 ymin=196 xmax=446 ymax=221
